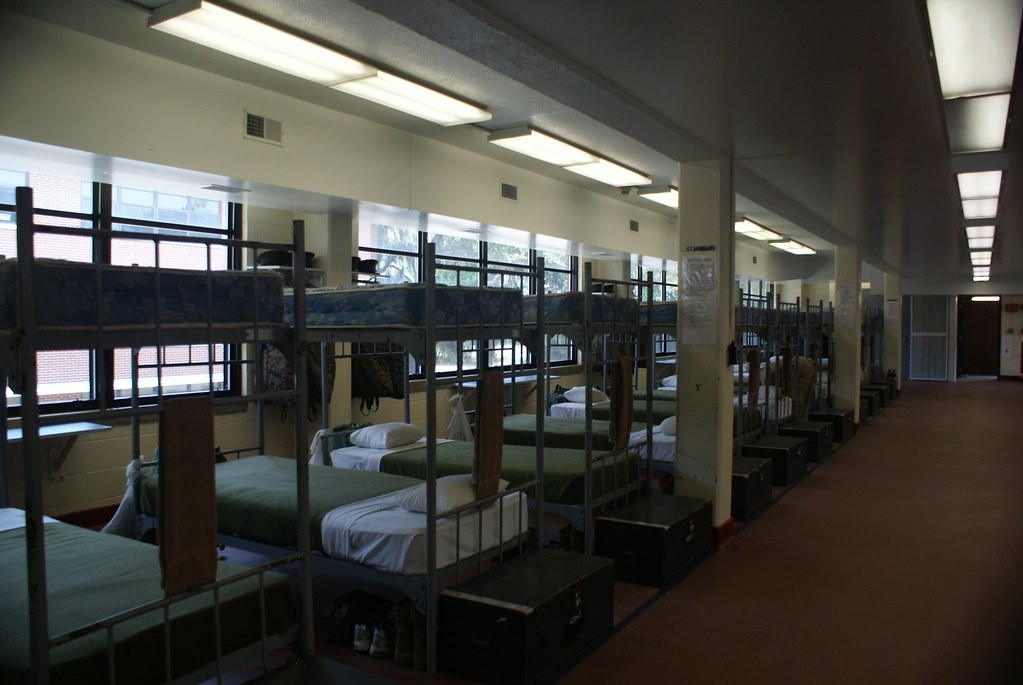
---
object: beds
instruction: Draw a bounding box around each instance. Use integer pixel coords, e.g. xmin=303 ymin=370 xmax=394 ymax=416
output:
xmin=0 ymin=187 xmax=884 ymax=685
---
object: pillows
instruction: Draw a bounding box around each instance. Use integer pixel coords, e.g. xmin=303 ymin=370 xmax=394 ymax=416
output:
xmin=662 ymin=373 xmax=677 ymax=388
xmin=392 ymin=474 xmax=509 ymax=517
xmin=661 ymin=415 xmax=676 ymax=436
xmin=349 ymin=422 xmax=425 ymax=448
xmin=564 ymin=386 xmax=609 ymax=403
xmin=747 ymin=386 xmax=781 ymax=401
xmin=771 ymin=355 xmax=783 ymax=363
xmin=733 ymin=363 xmax=751 ymax=373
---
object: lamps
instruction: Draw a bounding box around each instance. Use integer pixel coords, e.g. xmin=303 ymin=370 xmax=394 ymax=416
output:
xmin=767 ymin=239 xmax=817 ymax=255
xmin=734 ymin=217 xmax=784 ymax=241
xmin=486 ymin=125 xmax=652 ymax=187
xmin=147 ymin=0 xmax=492 ymax=128
xmin=920 ymin=0 xmax=1023 ymax=283
xmin=637 ymin=186 xmax=678 ymax=210
